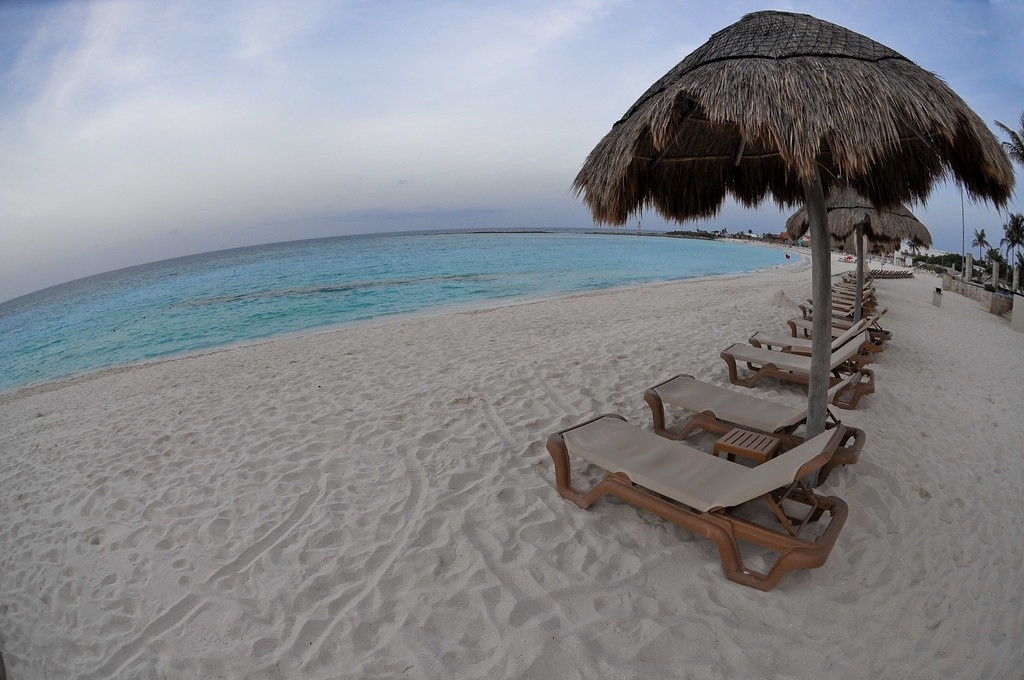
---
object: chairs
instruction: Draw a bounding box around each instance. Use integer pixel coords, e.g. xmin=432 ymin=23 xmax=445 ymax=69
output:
xmin=546 ymin=270 xmax=914 ymax=591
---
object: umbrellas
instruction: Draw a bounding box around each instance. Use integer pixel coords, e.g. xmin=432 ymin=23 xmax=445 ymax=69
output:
xmin=786 ymin=184 xmax=933 ymax=325
xmin=570 ymin=11 xmax=1019 ymax=489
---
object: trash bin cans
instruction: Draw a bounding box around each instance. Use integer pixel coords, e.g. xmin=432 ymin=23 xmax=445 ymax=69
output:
xmin=932 ymin=286 xmax=943 ymax=307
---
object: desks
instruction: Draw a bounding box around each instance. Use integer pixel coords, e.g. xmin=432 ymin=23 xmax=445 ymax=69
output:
xmin=714 ymin=428 xmax=782 ymax=463
